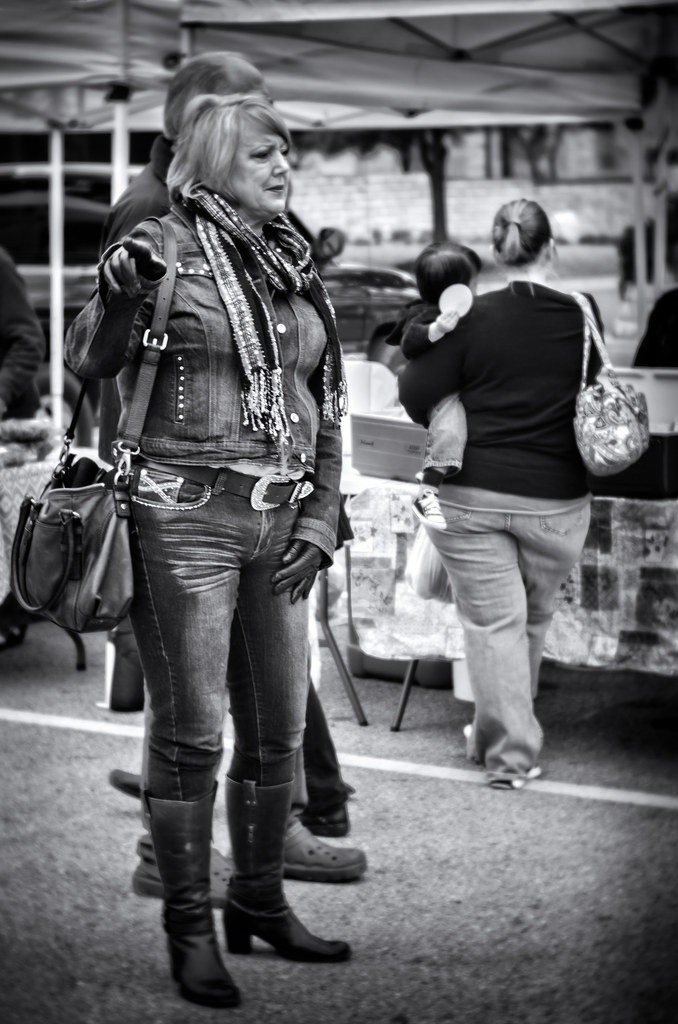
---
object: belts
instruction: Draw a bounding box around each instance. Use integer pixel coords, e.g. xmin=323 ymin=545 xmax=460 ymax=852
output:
xmin=138 ymin=460 xmax=317 ymax=511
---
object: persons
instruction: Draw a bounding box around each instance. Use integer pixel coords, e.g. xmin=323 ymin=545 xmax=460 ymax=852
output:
xmin=94 ymin=51 xmax=368 ymax=907
xmin=384 ymin=243 xmax=483 ymax=530
xmin=399 ymin=199 xmax=606 ymax=790
xmin=0 ymin=250 xmax=44 ymax=422
xmin=63 ymin=91 xmax=352 ymax=1009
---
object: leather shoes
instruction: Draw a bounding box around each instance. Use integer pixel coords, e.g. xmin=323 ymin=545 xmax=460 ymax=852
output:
xmin=294 ymin=809 xmax=350 ymax=837
xmin=108 ymin=769 xmax=144 ymax=800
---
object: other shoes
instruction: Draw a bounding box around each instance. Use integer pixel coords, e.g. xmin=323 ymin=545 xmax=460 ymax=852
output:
xmin=463 ymin=723 xmax=542 ymax=791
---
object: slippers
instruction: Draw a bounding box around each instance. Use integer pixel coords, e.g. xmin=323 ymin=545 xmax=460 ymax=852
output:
xmin=132 ymin=840 xmax=232 ymax=906
xmin=229 ymin=820 xmax=366 ymax=883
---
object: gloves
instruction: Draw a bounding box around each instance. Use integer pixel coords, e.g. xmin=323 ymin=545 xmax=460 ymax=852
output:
xmin=271 ymin=538 xmax=322 ymax=605
xmin=103 ymin=239 xmax=152 ymax=299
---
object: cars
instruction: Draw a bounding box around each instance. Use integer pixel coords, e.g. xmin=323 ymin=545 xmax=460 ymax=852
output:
xmin=1 ymin=191 xmax=119 ymax=351
xmin=313 ymin=261 xmax=422 ymax=346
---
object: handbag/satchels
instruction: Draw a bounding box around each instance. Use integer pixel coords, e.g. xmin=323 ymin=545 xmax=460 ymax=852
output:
xmin=10 ymin=454 xmax=133 ymax=634
xmin=568 ymin=291 xmax=650 ymax=476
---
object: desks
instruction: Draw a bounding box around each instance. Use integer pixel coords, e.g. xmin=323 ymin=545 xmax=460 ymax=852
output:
xmin=345 ymin=481 xmax=678 ymax=731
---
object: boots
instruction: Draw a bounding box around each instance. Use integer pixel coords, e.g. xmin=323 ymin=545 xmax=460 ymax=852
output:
xmin=150 ymin=781 xmax=243 ymax=1010
xmin=222 ymin=775 xmax=352 ymax=965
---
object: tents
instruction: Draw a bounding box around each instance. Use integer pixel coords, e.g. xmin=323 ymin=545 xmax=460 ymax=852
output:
xmin=1 ymin=0 xmax=678 ymax=458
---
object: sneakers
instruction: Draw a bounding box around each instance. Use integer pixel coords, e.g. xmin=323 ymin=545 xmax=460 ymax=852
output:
xmin=411 ymin=490 xmax=447 ymax=531
